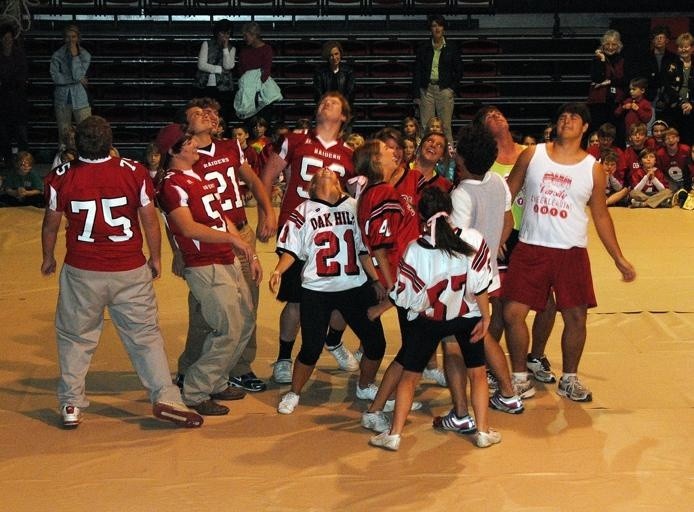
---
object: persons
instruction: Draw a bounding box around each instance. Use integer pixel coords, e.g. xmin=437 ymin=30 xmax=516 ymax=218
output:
xmin=39 ymin=91 xmax=636 ymax=450
xmin=190 ymin=18 xmax=237 ymax=136
xmin=411 ymin=12 xmax=463 ymax=148
xmin=635 ymin=25 xmax=675 ymax=120
xmin=587 ymin=121 xmax=694 ymax=209
xmin=586 ymin=28 xmax=626 ymax=108
xmin=614 ymin=76 xmax=652 ymax=143
xmin=662 ymin=32 xmax=692 ymax=145
xmin=47 ymin=26 xmax=92 ymax=151
xmin=238 ymin=21 xmax=283 ymax=133
xmin=0 ymin=151 xmax=45 ymax=206
xmin=311 ymin=38 xmax=356 ymax=134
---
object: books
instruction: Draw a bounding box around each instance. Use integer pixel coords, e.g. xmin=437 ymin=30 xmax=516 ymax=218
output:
xmin=587 ymin=84 xmax=608 ymax=104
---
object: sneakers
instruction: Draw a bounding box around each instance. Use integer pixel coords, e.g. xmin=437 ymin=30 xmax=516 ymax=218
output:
xmin=356 ymin=380 xmax=423 ymax=452
xmin=153 ymin=370 xmax=267 ymax=428
xmin=476 ymin=430 xmax=501 ymax=447
xmin=433 ymin=408 xmax=476 ymax=434
xmin=272 ymin=358 xmax=294 ymax=383
xmin=487 ymin=353 xmax=556 ymax=415
xmin=615 ymin=197 xmax=627 ymax=206
xmin=422 ymin=368 xmax=448 ymax=387
xmin=278 ymin=392 xmax=300 ymax=413
xmin=631 ymin=199 xmax=640 ymax=207
xmin=324 ymin=341 xmax=363 ymax=372
xmin=61 ymin=405 xmax=79 ymax=427
xmin=557 ymin=376 xmax=592 ymax=403
xmin=661 ymin=199 xmax=671 ymax=207
